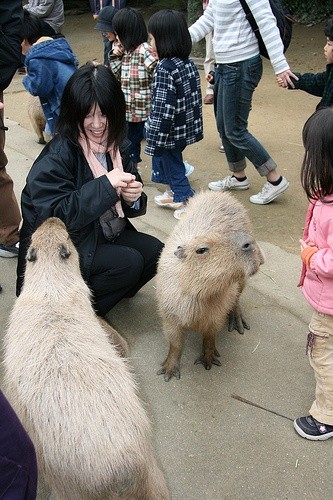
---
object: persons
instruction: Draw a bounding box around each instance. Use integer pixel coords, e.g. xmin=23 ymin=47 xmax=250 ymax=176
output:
xmin=186 ymin=0 xmax=298 ymax=205
xmin=17 ymin=16 xmax=79 ymax=139
xmin=16 ymin=61 xmax=165 ymax=318
xmin=90 ymin=0 xmax=226 ymax=177
xmin=18 ymin=0 xmax=65 ymax=73
xmin=0 ymin=0 xmax=23 ymax=258
xmin=277 ymin=27 xmax=333 ymax=110
xmin=293 ymin=108 xmax=333 ymax=441
xmin=147 ymin=9 xmax=204 ymax=221
xmin=108 ymin=7 xmax=194 ymax=177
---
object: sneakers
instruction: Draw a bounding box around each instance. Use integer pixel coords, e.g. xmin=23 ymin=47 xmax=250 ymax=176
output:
xmin=154 ymin=189 xmax=184 ymax=209
xmin=208 ymin=176 xmax=250 ymax=191
xmin=174 ymin=205 xmax=185 ymax=220
xmin=293 ymin=415 xmax=333 ymax=440
xmin=250 ymin=175 xmax=290 ymax=205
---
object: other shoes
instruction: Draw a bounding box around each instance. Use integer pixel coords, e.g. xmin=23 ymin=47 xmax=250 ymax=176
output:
xmin=204 ymin=94 xmax=214 ymax=103
xmin=1 ymin=241 xmax=20 ymax=258
xmin=219 ymin=145 xmax=226 ymax=153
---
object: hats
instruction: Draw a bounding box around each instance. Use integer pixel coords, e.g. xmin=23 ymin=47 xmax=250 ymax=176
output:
xmin=93 ymin=5 xmax=118 ymax=32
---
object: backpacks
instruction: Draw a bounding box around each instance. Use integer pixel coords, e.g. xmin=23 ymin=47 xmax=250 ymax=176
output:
xmin=239 ymin=0 xmax=292 ymax=60
